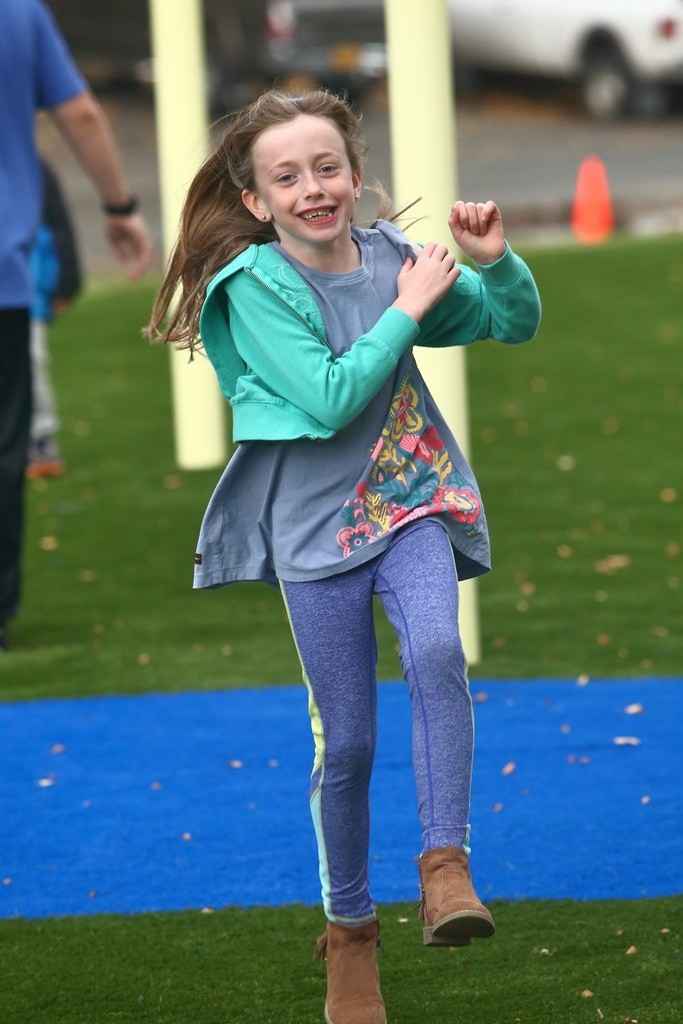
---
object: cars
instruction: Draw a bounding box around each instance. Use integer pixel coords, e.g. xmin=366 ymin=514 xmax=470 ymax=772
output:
xmin=89 ymin=1 xmax=384 ymax=116
xmin=448 ymin=0 xmax=683 ymax=126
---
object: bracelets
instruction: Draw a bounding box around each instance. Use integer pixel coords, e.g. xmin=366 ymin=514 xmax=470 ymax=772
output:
xmin=101 ymin=193 xmax=138 ymax=215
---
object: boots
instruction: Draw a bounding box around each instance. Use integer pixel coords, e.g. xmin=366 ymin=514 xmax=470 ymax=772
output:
xmin=413 ymin=848 xmax=496 ymax=946
xmin=315 ymin=920 xmax=387 ymax=1024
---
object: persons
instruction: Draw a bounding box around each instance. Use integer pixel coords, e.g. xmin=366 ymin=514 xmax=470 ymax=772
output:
xmin=140 ymin=92 xmax=541 ymax=1024
xmin=0 ymin=1 xmax=150 ymax=628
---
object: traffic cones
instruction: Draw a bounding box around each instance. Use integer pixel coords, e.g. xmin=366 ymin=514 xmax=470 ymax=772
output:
xmin=566 ymin=151 xmax=617 ymax=244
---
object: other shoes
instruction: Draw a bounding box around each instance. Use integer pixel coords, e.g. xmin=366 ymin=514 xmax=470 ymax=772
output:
xmin=23 ymin=437 xmax=62 ymax=477
